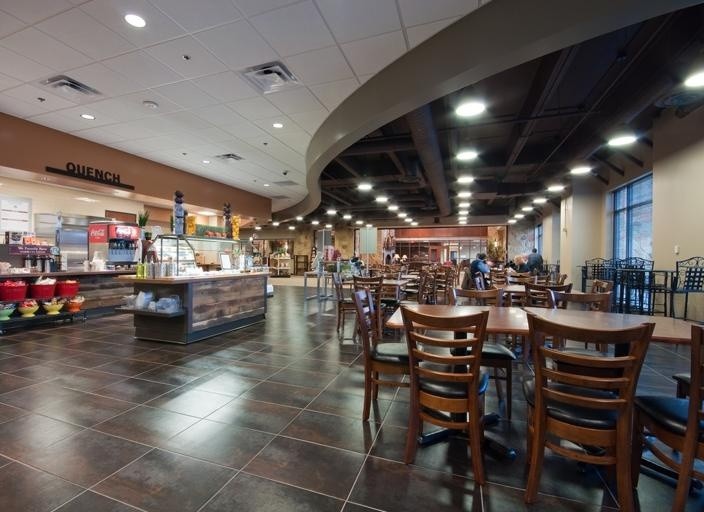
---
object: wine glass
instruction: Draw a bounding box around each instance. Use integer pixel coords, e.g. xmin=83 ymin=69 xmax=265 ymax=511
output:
xmin=0 ymin=295 xmax=86 ymax=321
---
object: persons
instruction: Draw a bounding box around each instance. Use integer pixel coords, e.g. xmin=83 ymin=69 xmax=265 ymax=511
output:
xmin=471 ymin=253 xmax=490 ymax=287
xmin=514 ymin=255 xmax=529 ymax=273
xmin=527 ymin=248 xmax=543 ymax=272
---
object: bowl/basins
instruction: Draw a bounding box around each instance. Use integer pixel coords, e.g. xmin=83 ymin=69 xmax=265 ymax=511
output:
xmin=0 ymin=280 xmax=80 ymax=300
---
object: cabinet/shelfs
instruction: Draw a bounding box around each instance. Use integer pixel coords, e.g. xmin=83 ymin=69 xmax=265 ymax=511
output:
xmin=108 ymin=232 xmax=271 ymax=346
xmin=0 ymin=294 xmax=88 ymax=336
xmin=269 ymin=252 xmax=291 ymax=278
xmin=294 ymin=254 xmax=308 ymax=275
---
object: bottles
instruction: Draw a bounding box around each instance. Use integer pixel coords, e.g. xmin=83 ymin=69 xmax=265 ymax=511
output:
xmin=108 ymin=240 xmax=135 ymax=261
xmin=24 ymin=253 xmax=54 ymax=272
xmin=136 ymin=256 xmax=175 ymax=280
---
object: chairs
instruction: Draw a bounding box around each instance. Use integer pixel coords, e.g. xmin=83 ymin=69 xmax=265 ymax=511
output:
xmin=303 ymin=257 xmax=703 ymax=344
xmin=352 ymin=292 xmax=423 ymax=421
xmin=450 ymin=286 xmax=516 ymax=418
xmin=524 ymin=313 xmax=656 ymax=512
xmin=634 ymin=324 xmax=703 ymax=511
xmin=399 ymin=303 xmax=489 ymax=485
